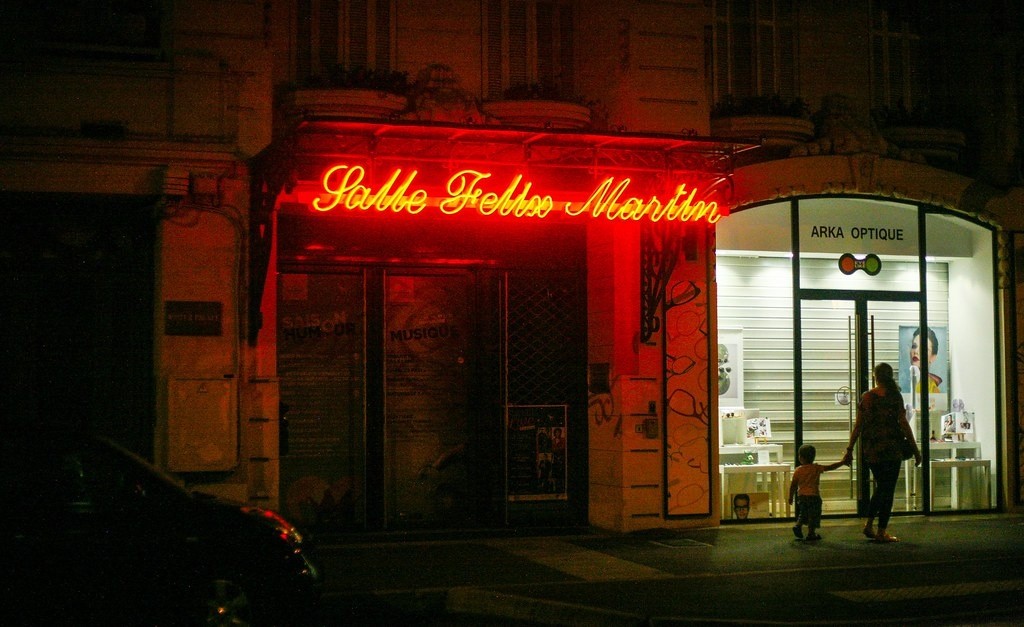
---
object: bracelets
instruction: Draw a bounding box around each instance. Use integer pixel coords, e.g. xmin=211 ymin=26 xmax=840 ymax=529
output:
xmin=847 ymin=447 xmax=853 ymax=451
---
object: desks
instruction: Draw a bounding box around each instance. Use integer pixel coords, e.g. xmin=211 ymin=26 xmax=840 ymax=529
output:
xmin=929 ymin=459 xmax=993 ymax=512
xmin=719 ymin=464 xmax=791 ymax=520
xmin=905 ymin=441 xmax=981 ymax=498
xmin=719 ymin=445 xmax=784 ymax=518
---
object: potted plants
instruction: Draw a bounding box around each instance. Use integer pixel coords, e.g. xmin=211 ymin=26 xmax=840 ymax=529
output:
xmin=861 ymin=109 xmax=986 ymax=170
xmin=709 ymin=83 xmax=818 ymax=142
xmin=274 ymin=56 xmax=416 ymax=119
xmin=470 ymin=81 xmax=612 ymax=129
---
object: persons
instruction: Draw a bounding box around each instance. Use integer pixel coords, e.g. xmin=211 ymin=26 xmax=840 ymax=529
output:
xmin=537 ymin=428 xmax=566 ymax=493
xmin=734 ymin=494 xmax=750 ymax=519
xmin=910 ymin=326 xmax=942 ymax=392
xmin=844 ymin=362 xmax=922 ymax=542
xmin=944 ymin=415 xmax=955 ymax=432
xmin=788 ymin=444 xmax=849 ymax=540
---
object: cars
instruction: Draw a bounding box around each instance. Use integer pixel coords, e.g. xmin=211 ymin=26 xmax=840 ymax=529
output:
xmin=0 ymin=410 xmax=322 ymax=627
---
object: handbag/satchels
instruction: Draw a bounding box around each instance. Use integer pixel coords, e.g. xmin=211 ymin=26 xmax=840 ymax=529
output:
xmin=902 ymin=439 xmax=914 ymax=460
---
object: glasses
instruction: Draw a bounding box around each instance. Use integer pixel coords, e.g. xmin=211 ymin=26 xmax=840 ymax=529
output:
xmin=735 ymin=506 xmax=750 ymax=511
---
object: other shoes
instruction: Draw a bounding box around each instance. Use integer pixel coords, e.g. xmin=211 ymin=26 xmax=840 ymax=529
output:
xmin=863 ymin=528 xmax=876 ymax=538
xmin=806 ymin=534 xmax=822 ymax=541
xmin=793 ymin=526 xmax=803 ymax=538
xmin=875 ymin=534 xmax=899 ymax=542
